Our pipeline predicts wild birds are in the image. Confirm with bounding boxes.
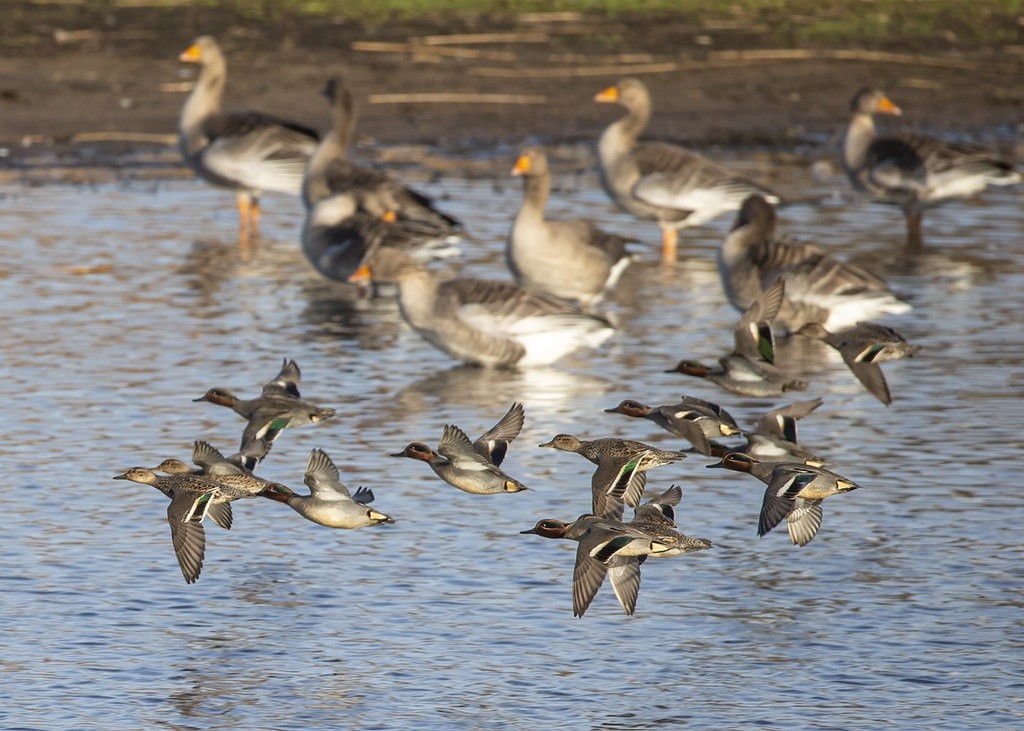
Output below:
[391,261,609,373]
[788,322,923,406]
[602,396,828,469]
[538,434,686,521]
[716,193,908,333]
[299,72,463,301]
[266,449,395,529]
[661,276,806,396]
[114,468,255,584]
[505,137,645,299]
[837,89,1020,248]
[150,442,266,530]
[705,451,861,548]
[594,80,782,265]
[192,358,334,462]
[390,401,536,495]
[171,34,326,235]
[606,484,714,617]
[517,515,673,618]
